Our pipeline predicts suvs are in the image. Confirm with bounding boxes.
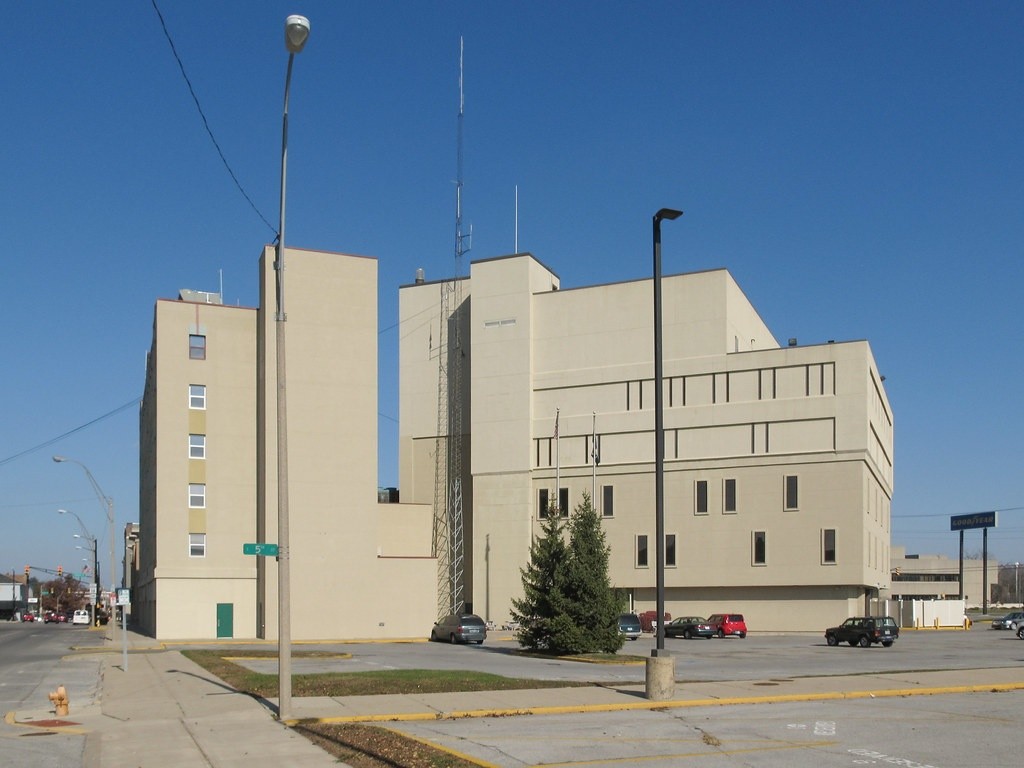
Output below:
[824,615,899,649]
[1001,612,1024,631]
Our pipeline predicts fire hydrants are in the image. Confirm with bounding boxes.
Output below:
[48,686,68,718]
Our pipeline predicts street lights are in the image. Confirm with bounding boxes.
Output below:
[52,454,117,645]
[57,508,98,631]
[638,206,684,685]
[276,14,313,727]
[1014,562,1020,603]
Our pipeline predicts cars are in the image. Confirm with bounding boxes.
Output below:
[1016,620,1024,641]
[34,611,69,625]
[616,612,643,641]
[431,614,487,644]
[23,613,34,622]
[665,617,719,639]
[991,617,1003,630]
[707,614,748,639]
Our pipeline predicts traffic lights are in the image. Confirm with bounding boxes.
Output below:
[57,566,62,576]
[24,566,29,576]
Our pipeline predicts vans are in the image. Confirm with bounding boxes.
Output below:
[72,609,90,626]
